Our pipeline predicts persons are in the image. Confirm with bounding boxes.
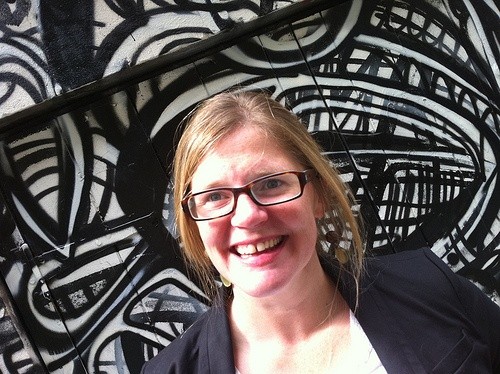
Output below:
[140,92,500,374]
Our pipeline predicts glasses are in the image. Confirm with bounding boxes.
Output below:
[180,168,319,220]
